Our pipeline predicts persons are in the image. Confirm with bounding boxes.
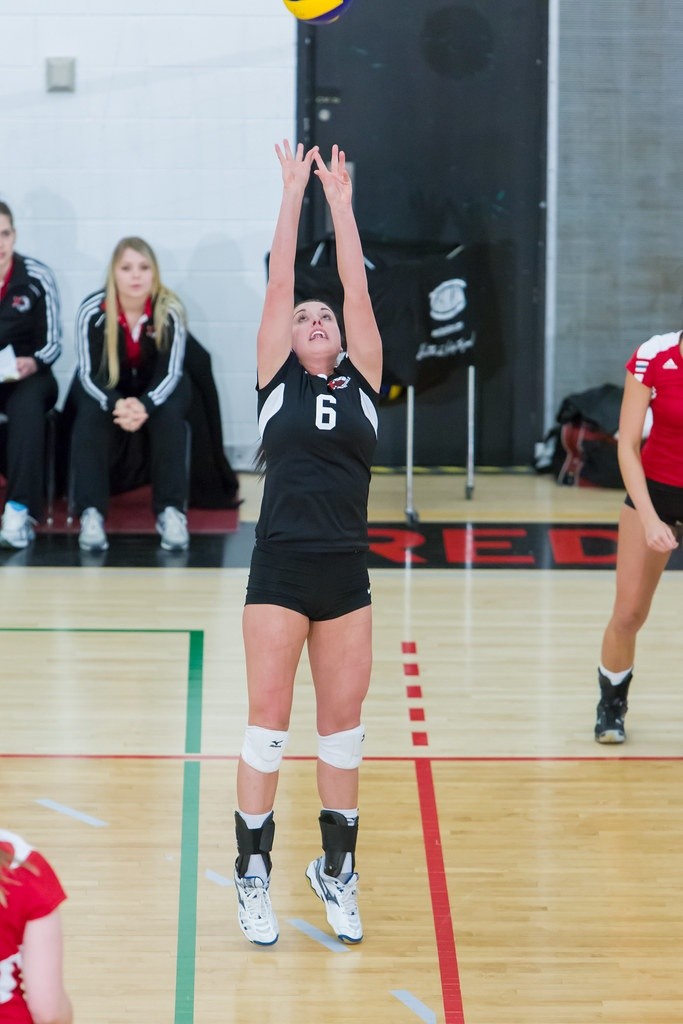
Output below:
[235,137,383,944]
[70,238,192,551]
[595,329,683,741]
[0,202,62,549]
[0,828,72,1024]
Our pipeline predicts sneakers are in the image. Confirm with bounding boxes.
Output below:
[233,865,280,946]
[594,700,628,744]
[0,501,37,549]
[304,855,364,945]
[155,506,190,553]
[78,506,110,553]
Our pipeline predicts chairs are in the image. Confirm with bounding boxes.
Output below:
[65,418,192,527]
[0,410,57,525]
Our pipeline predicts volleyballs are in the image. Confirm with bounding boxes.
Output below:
[280,0,353,28]
